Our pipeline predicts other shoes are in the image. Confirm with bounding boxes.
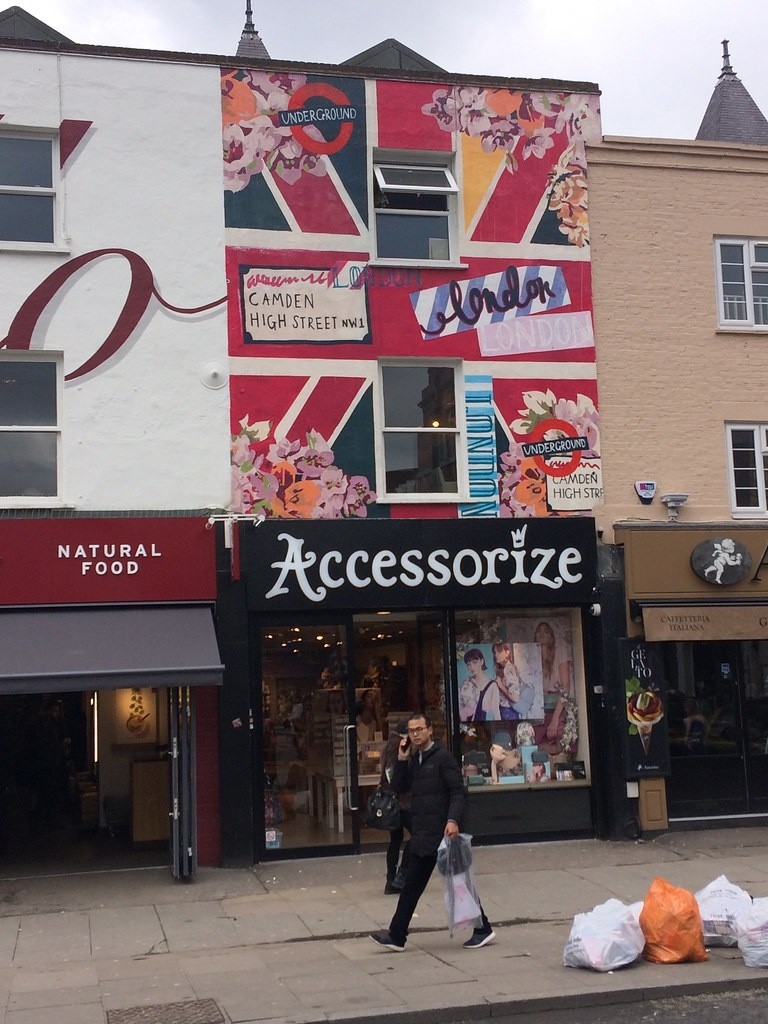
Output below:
[385,886,401,894]
[392,877,406,889]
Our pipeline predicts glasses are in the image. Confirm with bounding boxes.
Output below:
[406,727,428,734]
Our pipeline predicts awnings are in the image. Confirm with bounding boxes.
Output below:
[630,598,768,642]
[0,609,225,695]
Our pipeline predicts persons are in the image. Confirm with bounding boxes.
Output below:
[529,761,549,782]
[460,648,502,721]
[491,642,521,721]
[269,706,311,823]
[483,721,511,751]
[382,721,412,896]
[369,711,496,953]
[527,622,577,752]
[357,689,382,741]
[685,699,707,750]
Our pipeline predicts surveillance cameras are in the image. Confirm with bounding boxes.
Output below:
[588,603,601,617]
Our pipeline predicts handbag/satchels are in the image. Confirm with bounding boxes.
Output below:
[693,874,752,948]
[438,835,470,874]
[735,897,768,967]
[443,873,486,936]
[563,898,645,971]
[363,785,401,829]
[640,879,710,962]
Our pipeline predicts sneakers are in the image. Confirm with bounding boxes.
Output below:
[462,923,495,949]
[369,933,404,952]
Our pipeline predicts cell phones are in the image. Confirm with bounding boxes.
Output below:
[400,735,412,755]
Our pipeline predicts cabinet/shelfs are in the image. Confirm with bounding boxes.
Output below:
[269,688,442,833]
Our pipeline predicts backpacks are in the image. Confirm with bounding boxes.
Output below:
[508,682,535,714]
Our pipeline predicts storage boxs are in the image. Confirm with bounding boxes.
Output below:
[469,746,584,786]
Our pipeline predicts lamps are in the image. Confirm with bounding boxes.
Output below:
[660,492,691,519]
